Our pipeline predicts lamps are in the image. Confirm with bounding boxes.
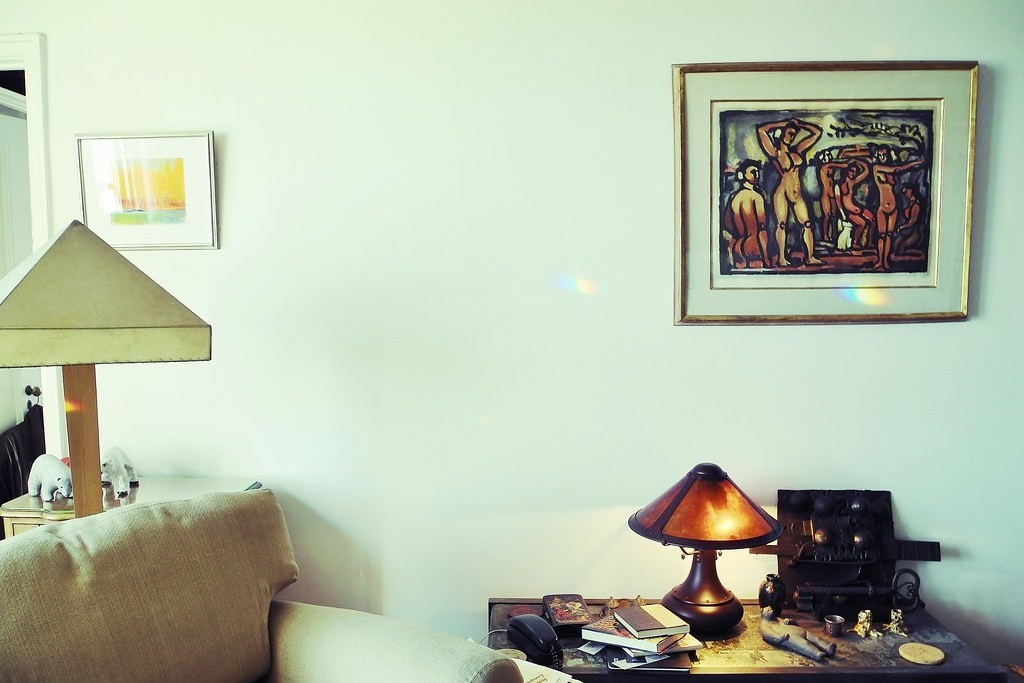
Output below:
[0,221,212,516]
[628,464,785,647]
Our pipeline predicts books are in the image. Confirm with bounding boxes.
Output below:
[619,627,703,658]
[613,603,690,640]
[604,641,694,672]
[580,615,689,656]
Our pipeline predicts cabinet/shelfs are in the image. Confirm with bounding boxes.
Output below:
[4,478,72,540]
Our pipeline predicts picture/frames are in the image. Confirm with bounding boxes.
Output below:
[671,57,981,331]
[73,132,224,254]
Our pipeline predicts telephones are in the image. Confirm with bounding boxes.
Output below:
[508,614,564,666]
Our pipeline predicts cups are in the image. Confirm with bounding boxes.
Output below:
[825,615,845,638]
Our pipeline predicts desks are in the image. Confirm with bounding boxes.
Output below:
[486,589,1011,683]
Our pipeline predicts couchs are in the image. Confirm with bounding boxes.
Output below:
[0,480,533,683]
